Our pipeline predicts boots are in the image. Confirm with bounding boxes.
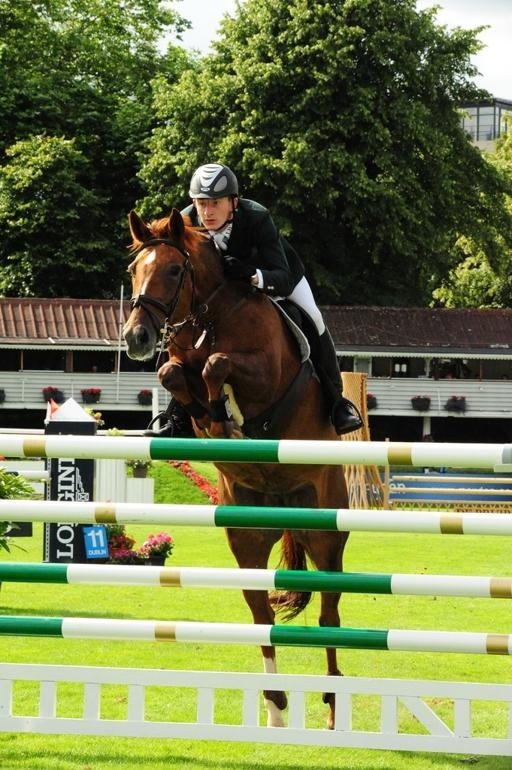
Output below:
[144,401,194,438]
[309,326,363,436]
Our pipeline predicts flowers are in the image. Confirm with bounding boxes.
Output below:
[108,525,176,564]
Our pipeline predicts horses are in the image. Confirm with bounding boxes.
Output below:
[122,207,351,730]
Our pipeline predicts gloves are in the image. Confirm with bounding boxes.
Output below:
[223,256,257,286]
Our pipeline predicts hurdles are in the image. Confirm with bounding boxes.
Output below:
[0,433,512,755]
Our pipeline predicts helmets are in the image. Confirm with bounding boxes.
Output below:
[188,162,239,200]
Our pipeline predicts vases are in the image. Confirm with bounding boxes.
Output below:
[145,555,166,566]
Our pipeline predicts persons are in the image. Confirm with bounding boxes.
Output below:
[140,163,362,439]
